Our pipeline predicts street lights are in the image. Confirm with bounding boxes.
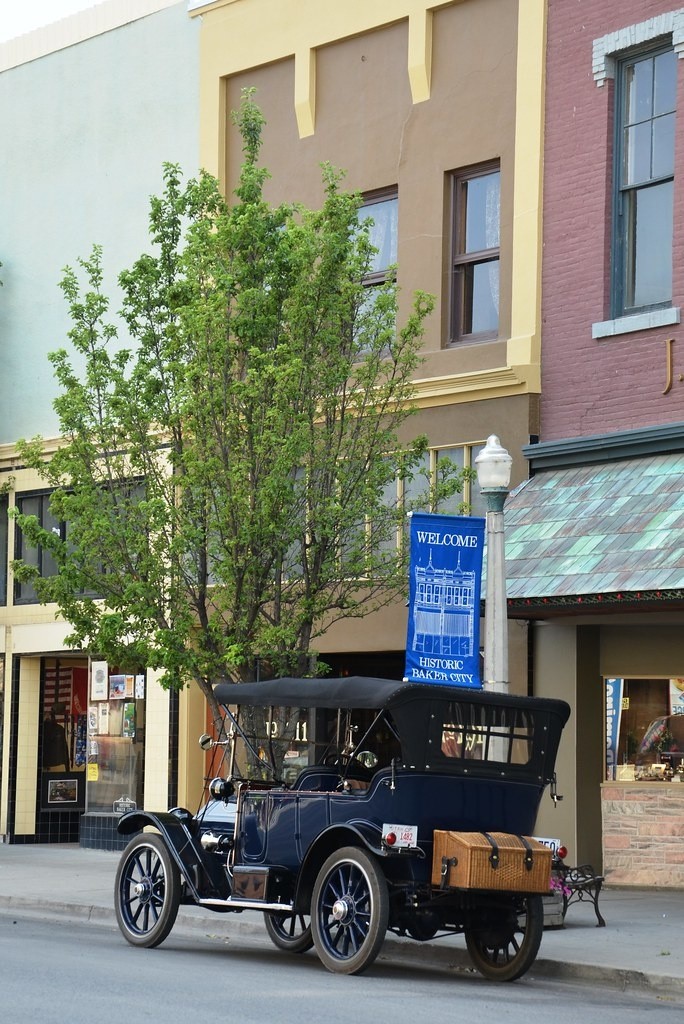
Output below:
[474,431,514,761]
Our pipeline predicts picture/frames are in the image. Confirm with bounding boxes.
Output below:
[48,780,78,803]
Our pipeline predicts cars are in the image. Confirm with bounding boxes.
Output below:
[113,675,572,983]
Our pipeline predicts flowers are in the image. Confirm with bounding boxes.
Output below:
[546,875,571,896]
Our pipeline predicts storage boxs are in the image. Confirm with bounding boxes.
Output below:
[432,828,553,894]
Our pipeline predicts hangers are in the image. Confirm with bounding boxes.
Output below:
[44,711,52,722]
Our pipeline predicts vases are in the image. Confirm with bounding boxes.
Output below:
[517,893,567,933]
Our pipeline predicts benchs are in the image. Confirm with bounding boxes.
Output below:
[290,765,377,792]
[551,857,606,930]
